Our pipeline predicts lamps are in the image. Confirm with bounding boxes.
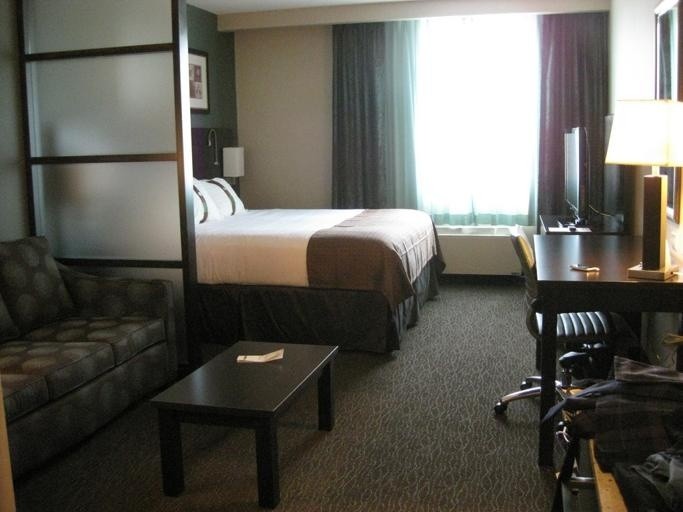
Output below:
[603,99,683,279]
[206,127,245,186]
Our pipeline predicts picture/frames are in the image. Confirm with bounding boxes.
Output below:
[187,47,210,114]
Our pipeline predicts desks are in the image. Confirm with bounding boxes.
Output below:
[524,233,683,468]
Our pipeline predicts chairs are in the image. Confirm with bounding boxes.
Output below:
[494,228,633,422]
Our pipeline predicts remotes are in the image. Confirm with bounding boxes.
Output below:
[568,225,576,231]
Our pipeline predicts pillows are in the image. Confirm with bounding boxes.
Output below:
[192,175,246,220]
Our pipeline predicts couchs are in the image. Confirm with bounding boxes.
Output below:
[0,235,180,484]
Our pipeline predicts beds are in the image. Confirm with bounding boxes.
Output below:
[192,209,445,355]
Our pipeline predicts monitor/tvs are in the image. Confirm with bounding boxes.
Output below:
[556,125,590,227]
